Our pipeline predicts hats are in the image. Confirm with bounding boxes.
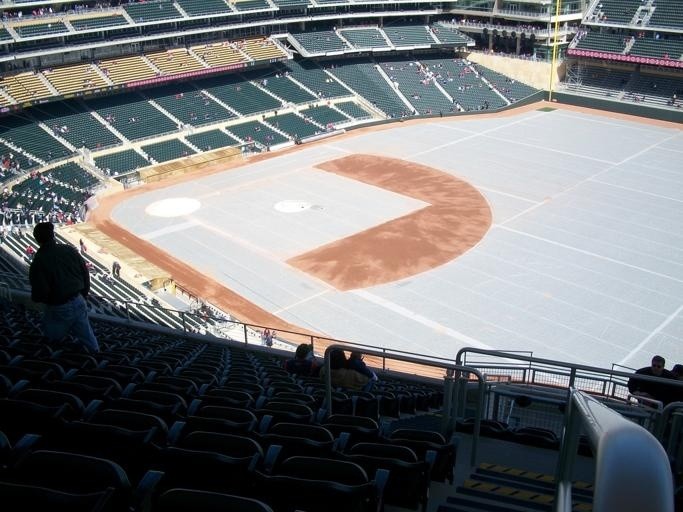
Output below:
[296,344,313,357]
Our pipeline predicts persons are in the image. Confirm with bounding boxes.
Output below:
[669,364,682,383]
[263,327,269,336]
[28,222,99,353]
[346,351,377,380]
[284,344,320,375]
[271,331,276,338]
[103,271,114,285]
[0,2,683,145]
[318,347,368,390]
[628,355,681,410]
[264,334,272,348]
[0,145,213,277]
